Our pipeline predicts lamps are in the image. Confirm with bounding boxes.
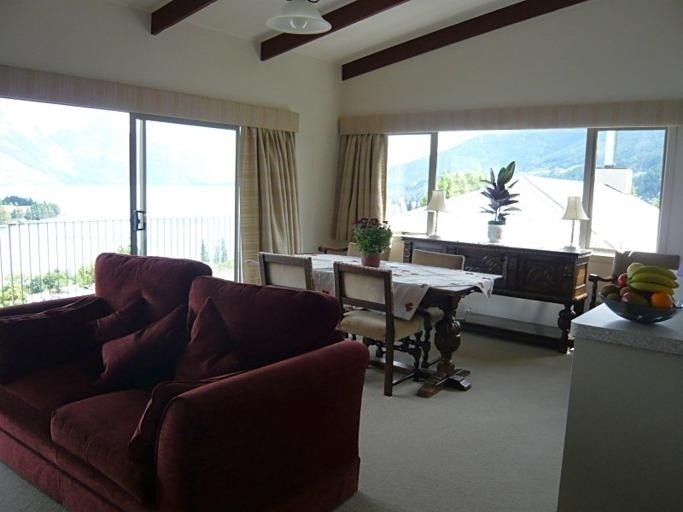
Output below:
[562,196,590,250]
[265,0,332,36]
[424,190,449,239]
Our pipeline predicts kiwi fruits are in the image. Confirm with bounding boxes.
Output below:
[619,287,632,297]
[600,285,619,297]
[607,293,619,303]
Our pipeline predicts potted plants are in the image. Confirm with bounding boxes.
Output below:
[352,218,391,267]
[479,161,521,244]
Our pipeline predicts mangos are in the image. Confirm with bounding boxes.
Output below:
[621,291,650,307]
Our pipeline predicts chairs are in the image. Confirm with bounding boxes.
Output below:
[348,242,404,262]
[588,250,680,309]
[376,250,465,368]
[258,252,315,293]
[100,303,188,391]
[81,298,143,373]
[333,261,425,396]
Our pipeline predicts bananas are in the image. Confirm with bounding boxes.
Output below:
[626,262,680,295]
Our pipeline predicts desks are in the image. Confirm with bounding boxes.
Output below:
[296,254,504,399]
[400,235,591,354]
[557,303,683,512]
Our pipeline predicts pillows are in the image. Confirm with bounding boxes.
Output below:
[176,297,244,379]
[126,370,246,462]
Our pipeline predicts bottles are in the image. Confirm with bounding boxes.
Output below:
[673,261,683,309]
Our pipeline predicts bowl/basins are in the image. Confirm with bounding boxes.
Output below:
[598,292,677,325]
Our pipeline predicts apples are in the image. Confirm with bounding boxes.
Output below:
[618,273,627,287]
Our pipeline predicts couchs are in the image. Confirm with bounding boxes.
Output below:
[0,253,369,511]
